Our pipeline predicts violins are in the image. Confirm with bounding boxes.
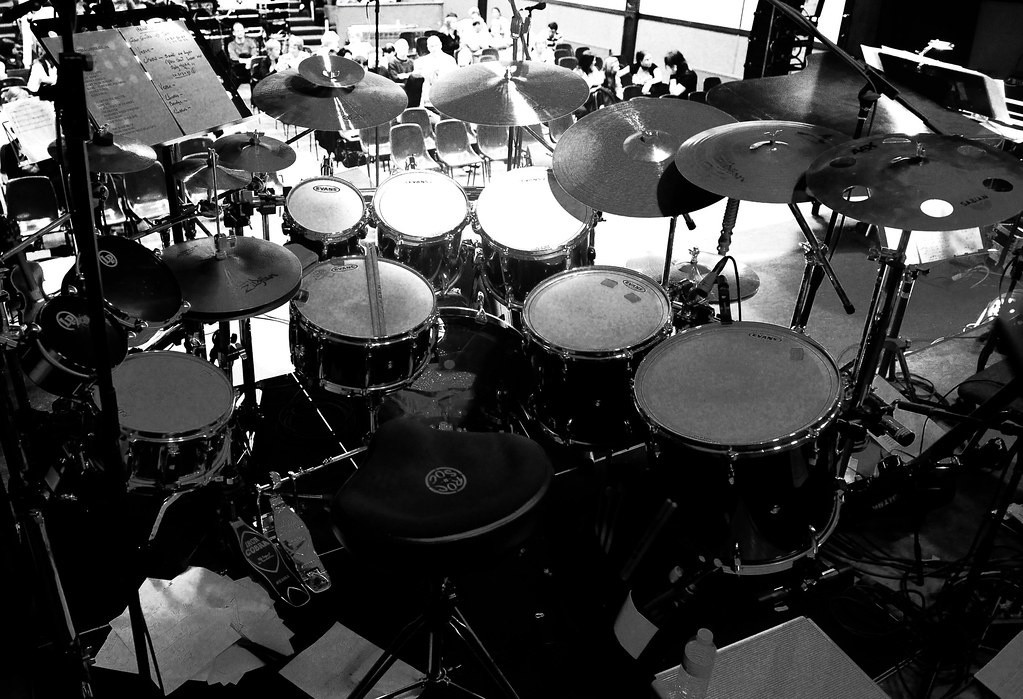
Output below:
[3,215,51,325]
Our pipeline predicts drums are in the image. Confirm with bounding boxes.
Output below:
[305,292,555,544]
[86,349,235,498]
[287,254,440,398]
[632,319,844,576]
[64,233,186,348]
[19,291,129,400]
[519,264,672,447]
[370,169,471,296]
[283,176,367,261]
[475,163,597,314]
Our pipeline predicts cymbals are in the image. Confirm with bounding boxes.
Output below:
[211,132,298,173]
[712,52,1006,150]
[546,95,740,219]
[49,131,159,175]
[805,129,1023,234]
[250,54,409,133]
[433,60,592,128]
[168,157,253,191]
[624,250,762,305]
[160,234,302,324]
[672,118,853,205]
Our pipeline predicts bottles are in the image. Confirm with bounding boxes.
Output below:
[675,628,717,699]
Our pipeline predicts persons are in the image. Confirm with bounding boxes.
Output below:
[5,31,57,103]
[228,7,565,89]
[572,50,697,100]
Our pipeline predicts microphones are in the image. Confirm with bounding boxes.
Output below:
[695,257,728,297]
[520,2,546,11]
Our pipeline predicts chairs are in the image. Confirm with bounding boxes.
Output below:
[0,31,720,240]
[974,628,1022,699]
